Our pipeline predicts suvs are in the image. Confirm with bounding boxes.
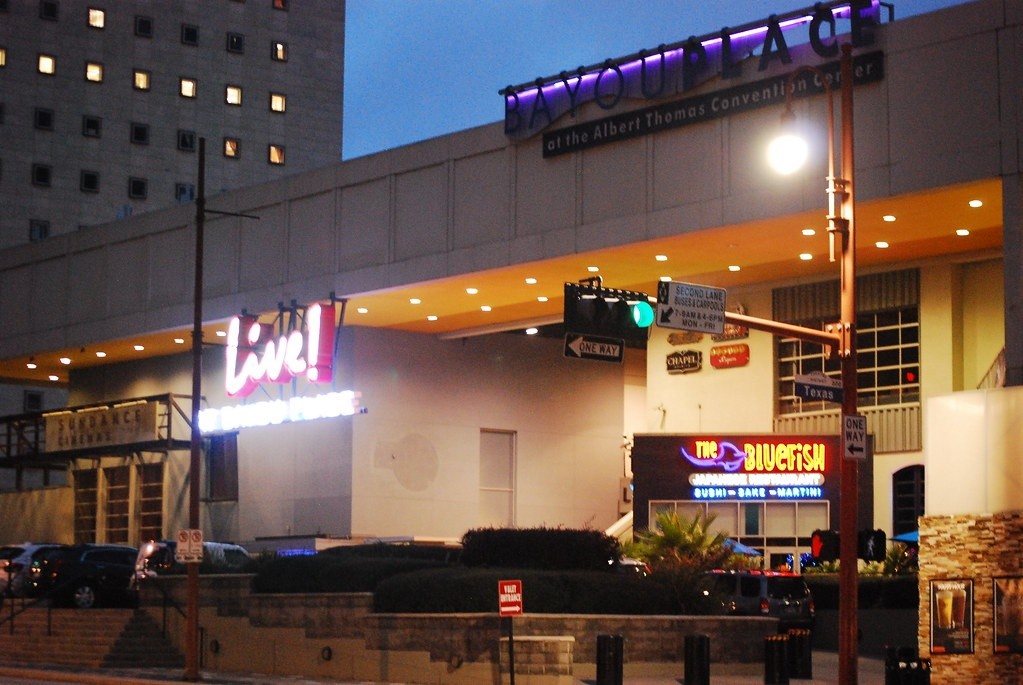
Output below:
[30,542,142,609]
[695,567,818,645]
[0,542,63,597]
[128,540,255,600]
[616,555,654,588]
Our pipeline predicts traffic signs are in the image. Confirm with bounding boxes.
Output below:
[498,579,523,617]
[561,331,626,363]
[654,279,726,334]
[841,414,868,460]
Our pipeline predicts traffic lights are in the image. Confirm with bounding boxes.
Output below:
[563,281,655,348]
[857,526,888,562]
[809,528,840,562]
